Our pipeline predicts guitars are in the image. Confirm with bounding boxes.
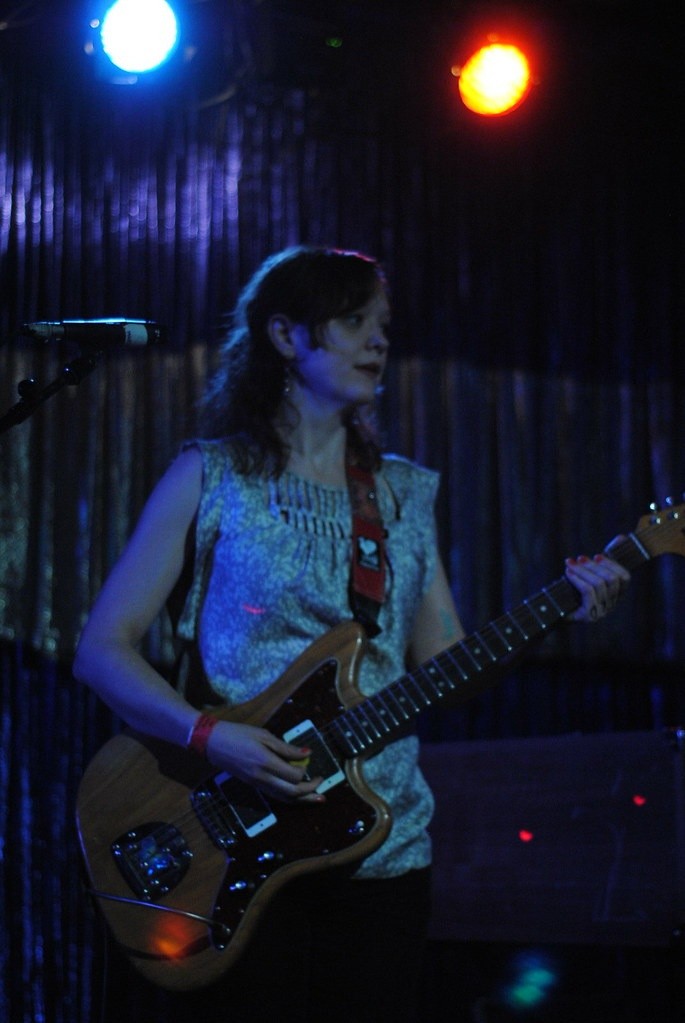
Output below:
[75,492,685,995]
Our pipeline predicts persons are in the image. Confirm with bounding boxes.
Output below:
[72,244,636,1023]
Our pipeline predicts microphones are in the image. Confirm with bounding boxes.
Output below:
[24,319,170,347]
[88,888,232,938]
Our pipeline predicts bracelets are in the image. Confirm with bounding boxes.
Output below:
[188,713,219,756]
